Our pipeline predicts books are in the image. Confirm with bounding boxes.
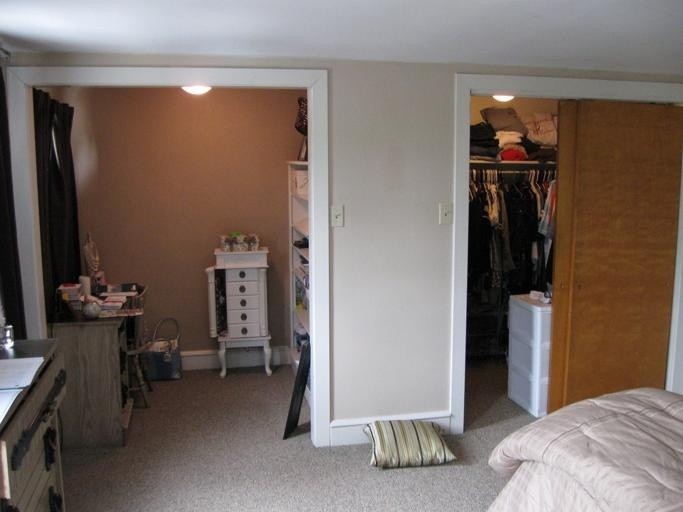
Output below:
[54,283,86,310]
[99,296,126,310]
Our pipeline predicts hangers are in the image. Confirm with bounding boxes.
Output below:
[468,167,555,199]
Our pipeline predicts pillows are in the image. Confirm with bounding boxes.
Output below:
[363,417,457,471]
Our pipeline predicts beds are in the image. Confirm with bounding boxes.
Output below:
[485,386,681,512]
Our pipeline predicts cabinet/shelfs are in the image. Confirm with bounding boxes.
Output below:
[283,159,309,401]
[53,281,138,446]
[205,248,275,379]
[0,330,68,511]
[505,290,550,419]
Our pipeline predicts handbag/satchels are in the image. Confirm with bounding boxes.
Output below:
[141,338,182,380]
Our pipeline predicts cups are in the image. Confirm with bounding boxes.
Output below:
[0,325,14,350]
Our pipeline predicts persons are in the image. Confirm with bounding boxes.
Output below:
[82,232,100,277]
